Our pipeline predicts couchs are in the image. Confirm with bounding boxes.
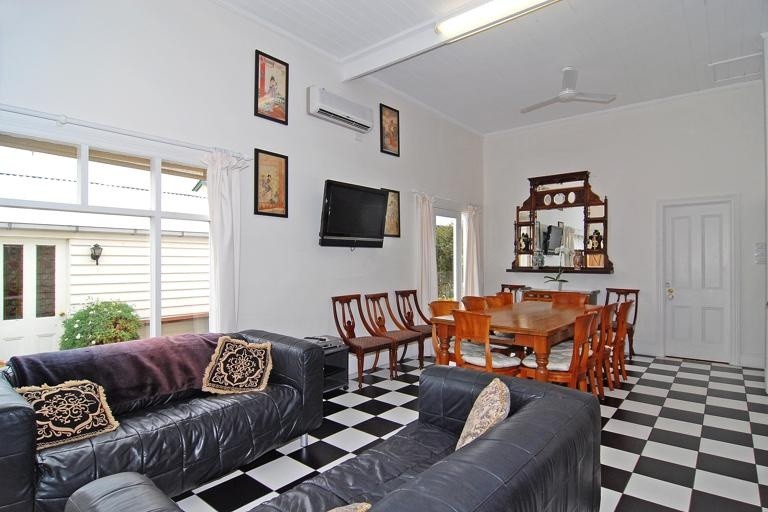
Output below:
[0,328,326,511]
[62,363,602,512]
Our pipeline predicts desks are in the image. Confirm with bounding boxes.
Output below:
[522,288,591,305]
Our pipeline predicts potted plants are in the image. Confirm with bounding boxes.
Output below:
[543,267,569,291]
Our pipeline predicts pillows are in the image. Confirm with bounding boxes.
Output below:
[326,500,374,512]
[11,378,120,452]
[454,377,512,453]
[200,335,273,395]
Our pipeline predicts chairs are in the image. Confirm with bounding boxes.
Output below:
[484,295,505,309]
[519,311,595,393]
[588,302,618,392]
[548,292,590,307]
[452,308,522,376]
[501,283,525,304]
[364,293,425,379]
[428,300,494,365]
[551,306,606,399]
[331,293,395,388]
[597,299,634,380]
[462,295,487,313]
[497,291,514,309]
[597,287,641,361]
[395,288,433,369]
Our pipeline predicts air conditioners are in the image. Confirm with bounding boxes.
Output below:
[306,84,373,135]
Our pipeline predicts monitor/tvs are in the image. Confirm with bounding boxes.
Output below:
[545,225,563,255]
[319,179,389,248]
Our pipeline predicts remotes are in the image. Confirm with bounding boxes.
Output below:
[304,335,326,342]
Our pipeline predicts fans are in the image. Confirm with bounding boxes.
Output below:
[519,67,617,115]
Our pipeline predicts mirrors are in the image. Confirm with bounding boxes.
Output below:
[506,169,616,274]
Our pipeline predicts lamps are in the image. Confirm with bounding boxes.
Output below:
[90,242,104,266]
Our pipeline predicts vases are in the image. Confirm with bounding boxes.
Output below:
[532,250,545,271]
[573,249,585,271]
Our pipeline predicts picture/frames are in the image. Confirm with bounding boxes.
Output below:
[254,48,290,128]
[378,102,402,158]
[253,147,289,219]
[380,187,402,240]
